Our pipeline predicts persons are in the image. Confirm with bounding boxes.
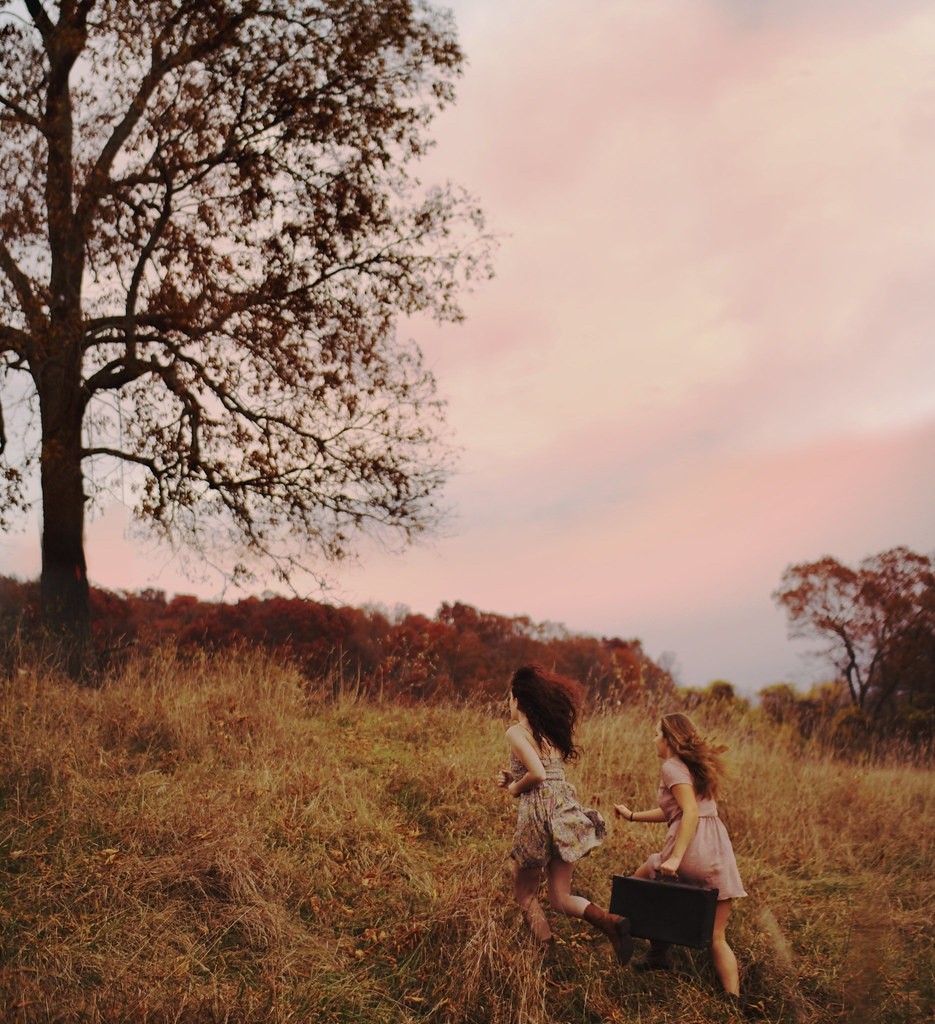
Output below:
[613,713,748,1009]
[497,665,632,988]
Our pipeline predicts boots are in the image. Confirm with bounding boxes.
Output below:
[539,935,562,965]
[584,902,634,967]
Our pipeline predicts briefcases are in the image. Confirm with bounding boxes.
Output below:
[609,869,720,950]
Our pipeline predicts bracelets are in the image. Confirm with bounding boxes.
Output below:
[631,812,634,822]
